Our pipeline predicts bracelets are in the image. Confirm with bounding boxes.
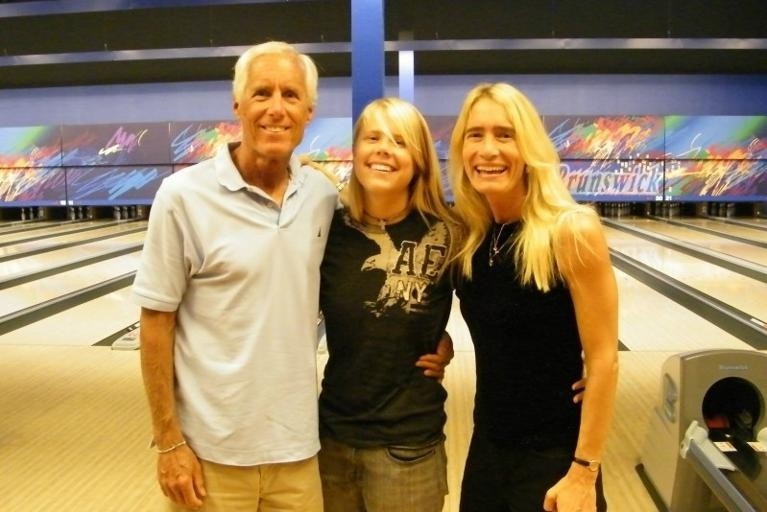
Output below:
[155,440,188,455]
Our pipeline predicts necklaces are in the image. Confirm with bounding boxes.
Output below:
[486,215,523,269]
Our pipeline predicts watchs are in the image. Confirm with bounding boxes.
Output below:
[571,455,600,472]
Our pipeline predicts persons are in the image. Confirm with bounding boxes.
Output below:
[316,98,589,512]
[442,82,622,512]
[130,39,455,512]
